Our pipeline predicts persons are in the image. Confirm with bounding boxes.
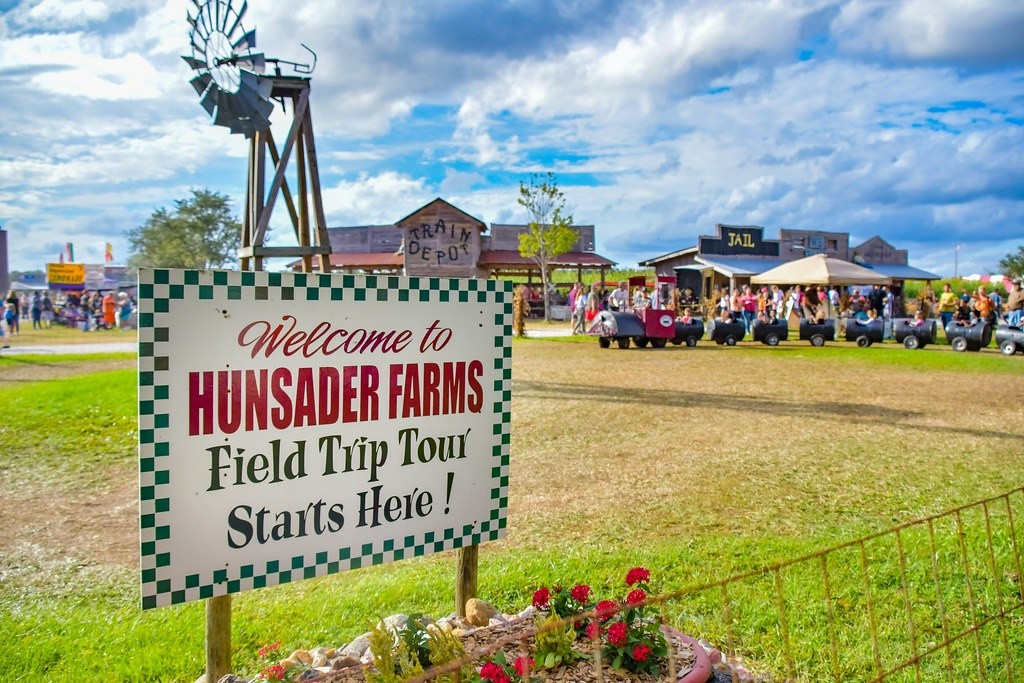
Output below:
[520,279,1022,348]
[0,289,132,339]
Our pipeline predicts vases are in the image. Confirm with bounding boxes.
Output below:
[305,618,711,683]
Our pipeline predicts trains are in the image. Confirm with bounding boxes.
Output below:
[584,304,1024,356]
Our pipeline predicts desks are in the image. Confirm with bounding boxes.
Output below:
[550,305,570,322]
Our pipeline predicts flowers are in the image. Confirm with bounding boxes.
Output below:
[255,567,668,683]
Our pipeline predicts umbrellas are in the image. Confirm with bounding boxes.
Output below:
[750,251,891,318]
